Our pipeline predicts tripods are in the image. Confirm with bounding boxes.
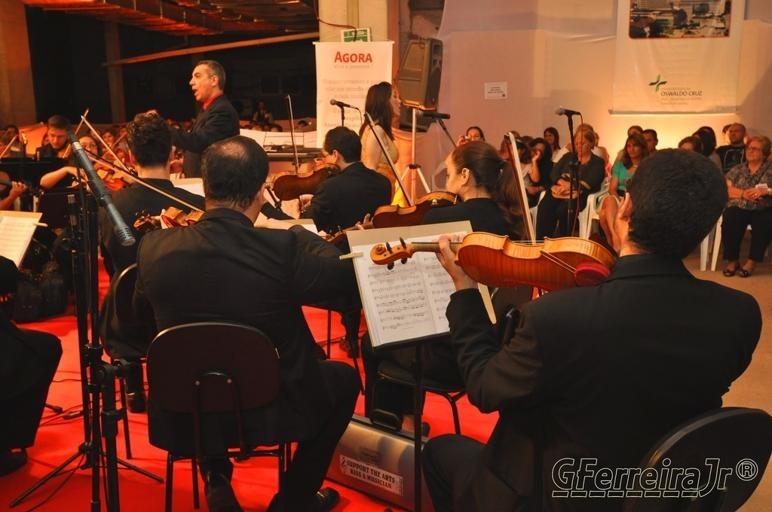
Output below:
[9,246,166,508]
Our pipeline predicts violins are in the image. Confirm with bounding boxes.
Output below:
[319,190,462,244]
[132,206,206,230]
[370,230,614,292]
[264,163,340,200]
[86,161,136,193]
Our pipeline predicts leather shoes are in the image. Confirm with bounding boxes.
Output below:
[738,267,751,276]
[206,471,244,512]
[0,452,27,475]
[265,487,339,510]
[123,389,146,412]
[723,268,736,276]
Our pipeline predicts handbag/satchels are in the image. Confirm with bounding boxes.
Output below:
[551,185,583,200]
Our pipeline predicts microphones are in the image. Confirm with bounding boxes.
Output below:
[330,99,356,111]
[555,108,580,117]
[416,109,452,120]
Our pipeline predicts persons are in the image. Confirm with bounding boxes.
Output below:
[453,120,772,279]
[260,126,393,358]
[357,80,403,203]
[128,131,364,510]
[359,139,522,437]
[669,2,689,30]
[420,147,763,512]
[0,99,313,317]
[1,255,65,479]
[142,57,242,178]
